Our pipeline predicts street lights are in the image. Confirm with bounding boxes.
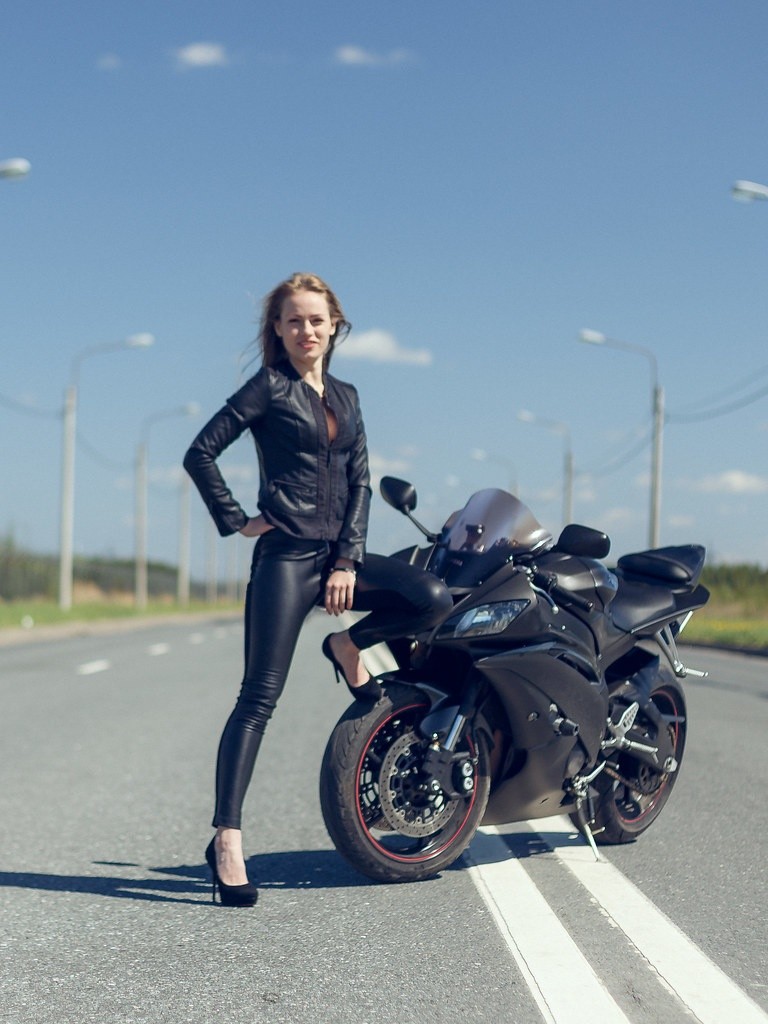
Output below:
[56,330,153,611]
[468,443,519,502]
[642,327,664,576]
[510,413,574,531]
[136,403,198,614]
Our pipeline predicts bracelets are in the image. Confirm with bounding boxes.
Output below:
[329,568,357,584]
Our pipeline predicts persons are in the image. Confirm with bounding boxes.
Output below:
[184,272,453,907]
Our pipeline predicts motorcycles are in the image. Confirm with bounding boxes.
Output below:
[315,476,714,884]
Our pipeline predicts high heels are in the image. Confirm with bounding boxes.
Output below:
[205,835,258,907]
[322,632,382,704]
